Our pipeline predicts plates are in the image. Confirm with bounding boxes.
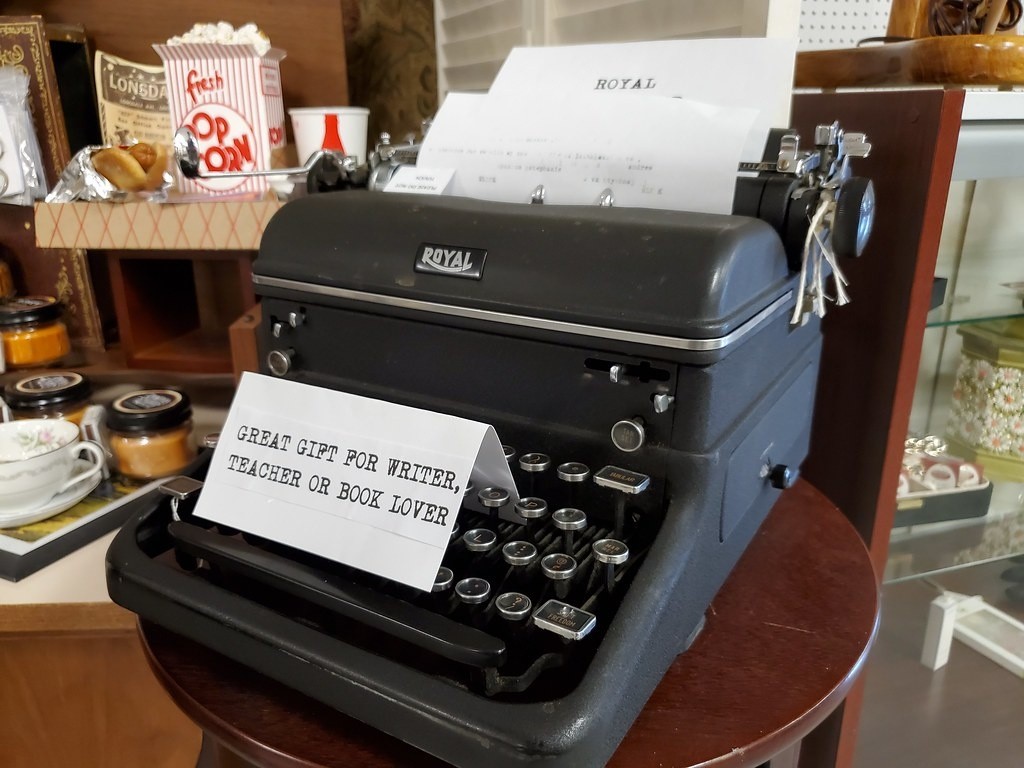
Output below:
[0,458,103,529]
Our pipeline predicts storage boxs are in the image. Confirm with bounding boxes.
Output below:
[150,42,289,195]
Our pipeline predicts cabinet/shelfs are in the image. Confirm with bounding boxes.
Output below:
[790,86,1024,587]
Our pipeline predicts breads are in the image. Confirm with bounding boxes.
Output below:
[91,142,168,193]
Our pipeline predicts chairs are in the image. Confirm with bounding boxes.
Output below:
[136,84,968,768]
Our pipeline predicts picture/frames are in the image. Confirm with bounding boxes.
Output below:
[0,472,183,582]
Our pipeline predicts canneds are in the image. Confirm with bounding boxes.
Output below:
[0,296,198,482]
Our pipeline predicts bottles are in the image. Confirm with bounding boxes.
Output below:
[0,293,198,489]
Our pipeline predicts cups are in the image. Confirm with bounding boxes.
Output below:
[0,420,106,514]
[287,106,371,169]
